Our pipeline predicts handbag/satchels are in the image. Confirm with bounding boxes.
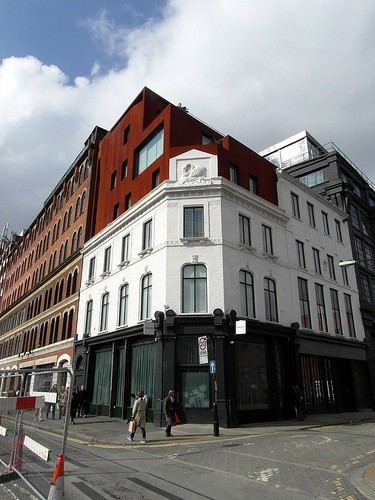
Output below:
[175,414,180,424]
[128,418,135,433]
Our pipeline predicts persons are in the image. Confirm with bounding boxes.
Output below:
[7,383,98,424]
[127,391,146,444]
[123,393,151,424]
[163,390,175,437]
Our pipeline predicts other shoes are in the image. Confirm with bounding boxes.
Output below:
[141,441,145,443]
[34,419,37,421]
[127,437,132,441]
[167,435,173,437]
[39,418,42,422]
